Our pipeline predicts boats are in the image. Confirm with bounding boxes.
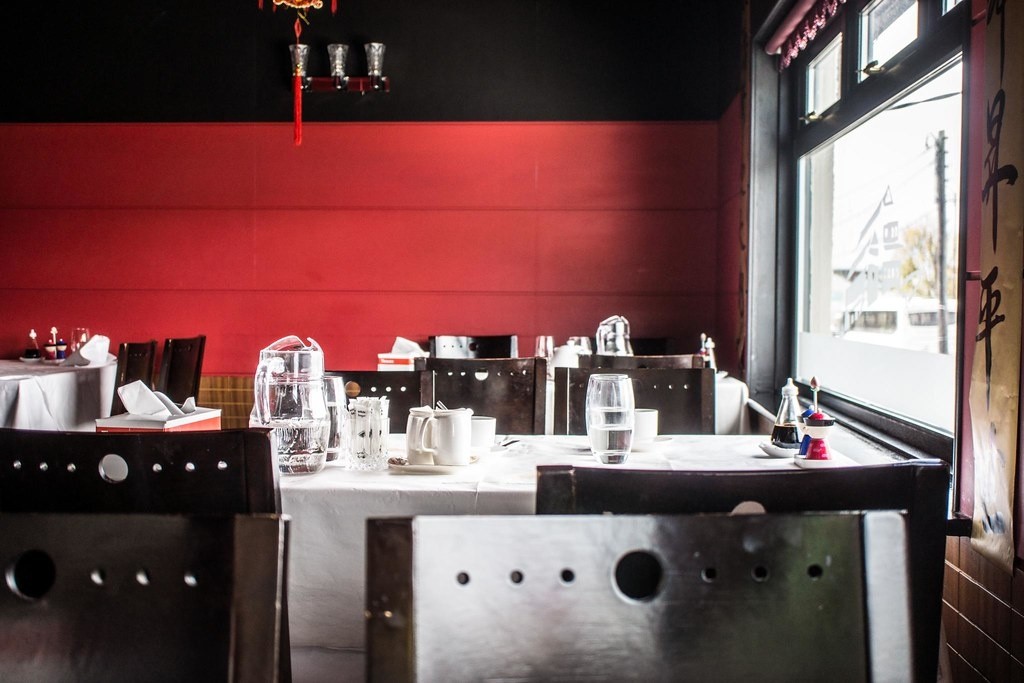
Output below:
[833,185,957,351]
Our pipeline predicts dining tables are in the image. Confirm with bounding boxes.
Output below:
[279,434,903,683]
[0,358,118,431]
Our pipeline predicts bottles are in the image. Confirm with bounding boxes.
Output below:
[770,377,806,449]
[24,328,41,358]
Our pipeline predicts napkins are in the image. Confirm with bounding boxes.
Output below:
[59,334,111,369]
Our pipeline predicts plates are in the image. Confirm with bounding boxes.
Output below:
[759,441,802,456]
[20,356,42,363]
[43,357,65,364]
[632,435,673,452]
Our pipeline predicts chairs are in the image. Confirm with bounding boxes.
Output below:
[325,332,752,432]
[109,340,158,416]
[156,333,207,408]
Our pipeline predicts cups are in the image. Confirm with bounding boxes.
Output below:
[71,328,89,352]
[535,330,592,381]
[469,415,497,449]
[585,373,657,463]
[405,407,436,464]
[318,372,347,462]
[342,412,391,471]
[419,405,473,466]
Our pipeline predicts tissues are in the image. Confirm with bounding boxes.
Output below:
[378,336,432,372]
[94,380,222,432]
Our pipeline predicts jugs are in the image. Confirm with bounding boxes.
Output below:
[255,334,331,476]
[596,313,637,355]
